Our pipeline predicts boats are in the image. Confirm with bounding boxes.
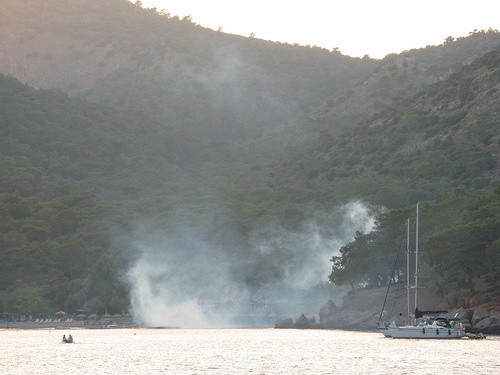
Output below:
[375,203,466,338]
